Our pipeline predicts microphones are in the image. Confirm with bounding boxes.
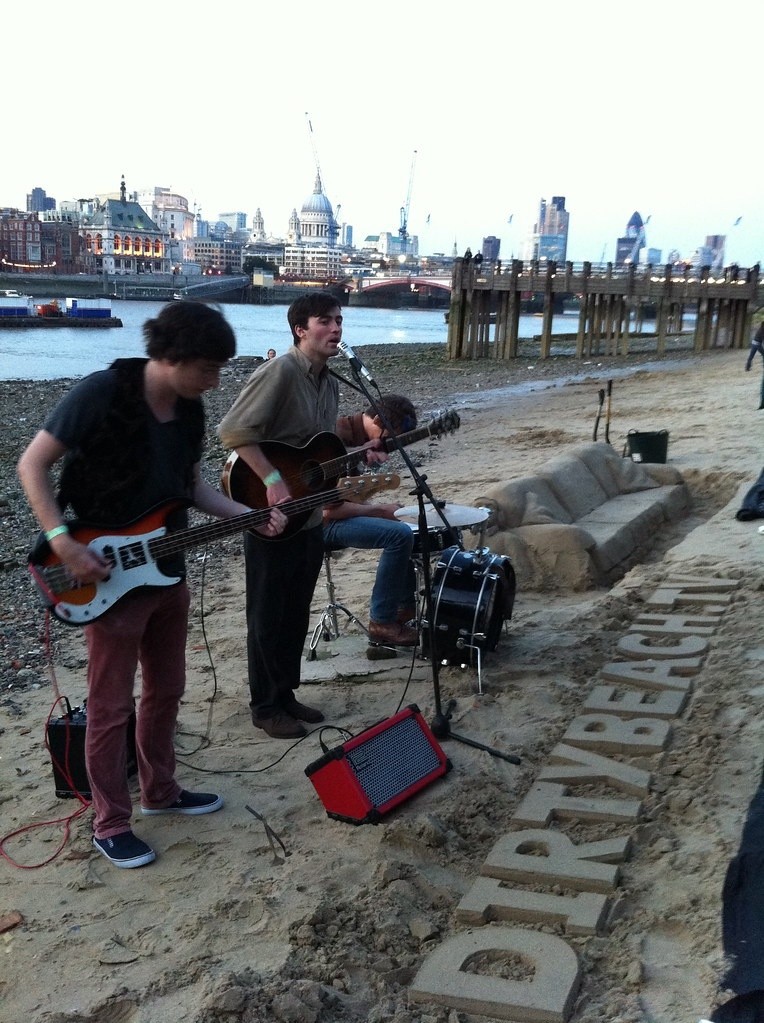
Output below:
[336,341,378,389]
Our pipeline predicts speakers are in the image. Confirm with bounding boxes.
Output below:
[47,704,139,800]
[304,703,454,826]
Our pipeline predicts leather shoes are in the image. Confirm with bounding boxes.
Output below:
[369,621,420,646]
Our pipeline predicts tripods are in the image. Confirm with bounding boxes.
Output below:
[351,368,522,765]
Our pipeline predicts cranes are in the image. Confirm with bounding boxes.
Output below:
[304,110,343,221]
[398,150,419,255]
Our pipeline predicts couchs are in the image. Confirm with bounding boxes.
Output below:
[474,442,688,588]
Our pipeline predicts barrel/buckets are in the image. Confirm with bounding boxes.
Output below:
[626,428,669,464]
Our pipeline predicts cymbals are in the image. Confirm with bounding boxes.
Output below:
[393,502,489,528]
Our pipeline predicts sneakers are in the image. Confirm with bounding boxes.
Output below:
[94,832,156,867]
[142,789,223,816]
[281,699,324,723]
[253,710,309,737]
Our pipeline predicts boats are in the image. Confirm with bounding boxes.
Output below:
[3,289,34,299]
[0,295,124,329]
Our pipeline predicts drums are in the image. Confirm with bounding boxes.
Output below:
[400,520,462,557]
[419,544,517,669]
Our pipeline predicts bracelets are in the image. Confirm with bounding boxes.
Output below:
[264,471,281,486]
[45,524,69,541]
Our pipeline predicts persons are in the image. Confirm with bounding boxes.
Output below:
[746,324,764,409]
[323,394,419,646]
[19,300,287,865]
[218,293,388,738]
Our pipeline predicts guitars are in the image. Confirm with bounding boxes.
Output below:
[223,406,464,541]
[25,468,401,627]
